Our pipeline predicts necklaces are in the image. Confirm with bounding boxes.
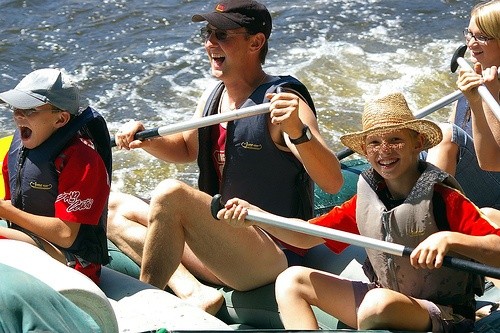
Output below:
[223,74,267,111]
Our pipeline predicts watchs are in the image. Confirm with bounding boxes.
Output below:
[288,125,313,145]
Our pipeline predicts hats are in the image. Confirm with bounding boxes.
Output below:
[340,92,443,156]
[191,0,272,39]
[0,67,80,117]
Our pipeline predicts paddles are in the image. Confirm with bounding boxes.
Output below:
[110,86,287,148]
[336,86,463,163]
[449,43,499,123]
[209,193,500,281]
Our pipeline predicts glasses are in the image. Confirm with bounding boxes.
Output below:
[462,27,496,43]
[199,26,251,40]
[8,101,63,115]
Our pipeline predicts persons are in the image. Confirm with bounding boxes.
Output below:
[475,207,500,321]
[419,0,500,210]
[0,68,110,285]
[456,62,500,173]
[216,93,500,333]
[106,0,343,317]
